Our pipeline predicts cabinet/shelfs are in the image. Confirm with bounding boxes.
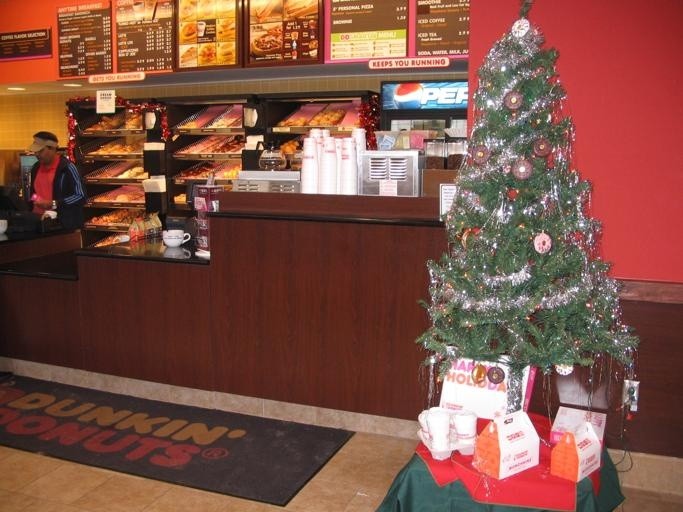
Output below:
[69,91,381,248]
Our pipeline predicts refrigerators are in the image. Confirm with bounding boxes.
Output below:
[380,79,468,137]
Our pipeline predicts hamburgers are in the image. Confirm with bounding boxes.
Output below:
[219,43,234,61]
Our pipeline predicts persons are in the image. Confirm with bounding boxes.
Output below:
[26,130,87,230]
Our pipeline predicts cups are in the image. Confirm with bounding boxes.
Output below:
[417,403,477,459]
[133,1,145,11]
[301,127,366,195]
[162,230,191,244]
[197,22,205,37]
[163,235,184,248]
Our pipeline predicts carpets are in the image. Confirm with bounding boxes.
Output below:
[0,367,354,506]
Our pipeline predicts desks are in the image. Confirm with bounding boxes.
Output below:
[412,412,617,511]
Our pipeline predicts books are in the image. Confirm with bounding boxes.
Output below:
[520,362,537,413]
[436,351,528,419]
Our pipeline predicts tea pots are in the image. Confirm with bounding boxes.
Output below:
[254,140,287,170]
[290,149,301,170]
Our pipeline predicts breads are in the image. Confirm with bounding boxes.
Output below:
[86,108,346,246]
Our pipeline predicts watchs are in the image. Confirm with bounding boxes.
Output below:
[50,199,57,211]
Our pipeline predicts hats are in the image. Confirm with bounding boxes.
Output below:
[26,136,58,152]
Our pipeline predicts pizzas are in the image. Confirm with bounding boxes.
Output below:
[252,34,282,56]
